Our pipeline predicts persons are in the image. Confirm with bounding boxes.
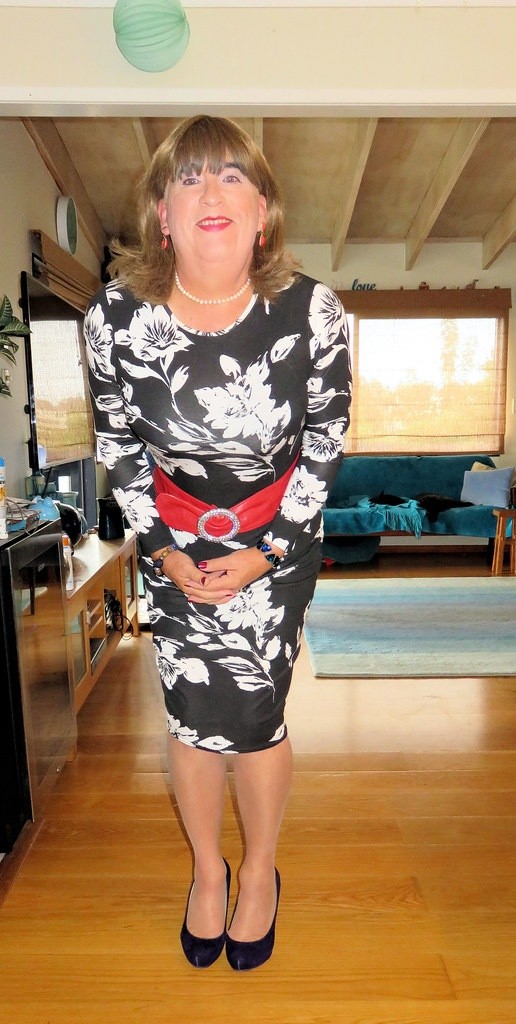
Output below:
[83,117,351,971]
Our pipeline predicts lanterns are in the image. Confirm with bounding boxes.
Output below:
[113,0,191,73]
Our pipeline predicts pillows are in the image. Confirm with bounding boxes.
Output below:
[470,460,495,470]
[460,466,516,509]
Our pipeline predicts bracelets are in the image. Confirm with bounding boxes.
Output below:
[256,539,281,570]
[154,542,178,577]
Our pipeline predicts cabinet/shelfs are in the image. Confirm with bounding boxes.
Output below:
[0,519,79,822]
[65,531,140,712]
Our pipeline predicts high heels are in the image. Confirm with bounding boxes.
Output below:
[180,856,231,967]
[226,866,281,970]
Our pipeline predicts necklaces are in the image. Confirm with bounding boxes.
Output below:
[171,262,252,304]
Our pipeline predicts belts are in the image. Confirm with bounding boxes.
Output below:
[152,447,300,541]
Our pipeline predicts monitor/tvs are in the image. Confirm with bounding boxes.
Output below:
[17,271,98,470]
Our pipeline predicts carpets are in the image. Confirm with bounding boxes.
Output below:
[306,577,516,678]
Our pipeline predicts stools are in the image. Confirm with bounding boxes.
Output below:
[491,509,516,576]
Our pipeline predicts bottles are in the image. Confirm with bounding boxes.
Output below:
[0,455,7,506]
[62,536,74,590]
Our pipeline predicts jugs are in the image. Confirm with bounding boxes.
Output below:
[96,497,126,540]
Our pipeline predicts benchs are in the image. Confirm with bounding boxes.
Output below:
[322,452,516,538]
